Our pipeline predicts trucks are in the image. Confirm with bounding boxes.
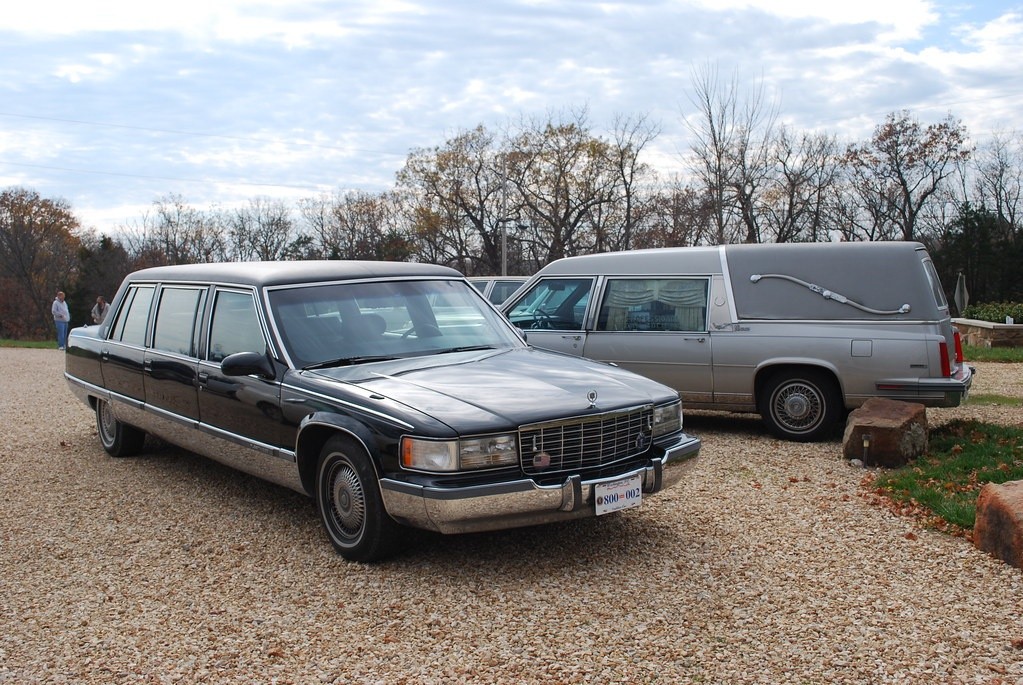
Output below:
[307,276,582,332]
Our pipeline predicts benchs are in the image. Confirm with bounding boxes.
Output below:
[278,317,343,345]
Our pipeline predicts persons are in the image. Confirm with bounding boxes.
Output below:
[52,292,70,350]
[91,296,112,325]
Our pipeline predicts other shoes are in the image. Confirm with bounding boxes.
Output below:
[59,346,67,349]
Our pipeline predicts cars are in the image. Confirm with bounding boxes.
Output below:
[63,260,701,563]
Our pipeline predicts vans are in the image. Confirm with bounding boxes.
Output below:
[381,241,976,443]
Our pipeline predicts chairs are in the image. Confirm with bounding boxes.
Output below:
[335,314,397,355]
